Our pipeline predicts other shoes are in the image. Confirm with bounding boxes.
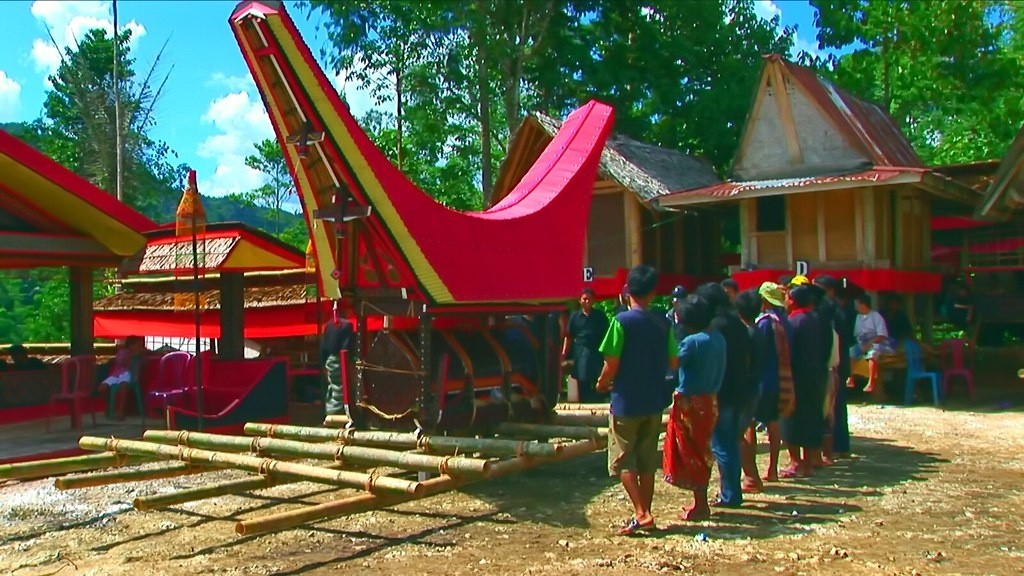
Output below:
[708,491,744,508]
[777,453,833,478]
[740,475,763,493]
[762,472,778,482]
[678,506,710,520]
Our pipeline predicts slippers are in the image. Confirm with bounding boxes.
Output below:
[615,511,656,535]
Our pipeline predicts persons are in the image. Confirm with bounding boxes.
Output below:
[99,334,148,421]
[321,308,357,417]
[561,263,974,537]
[9,345,49,405]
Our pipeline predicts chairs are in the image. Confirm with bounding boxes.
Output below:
[46,350,188,433]
[904,337,944,406]
[938,340,979,404]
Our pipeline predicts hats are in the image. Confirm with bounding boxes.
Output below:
[757,281,785,307]
[671,286,688,302]
[791,275,810,286]
